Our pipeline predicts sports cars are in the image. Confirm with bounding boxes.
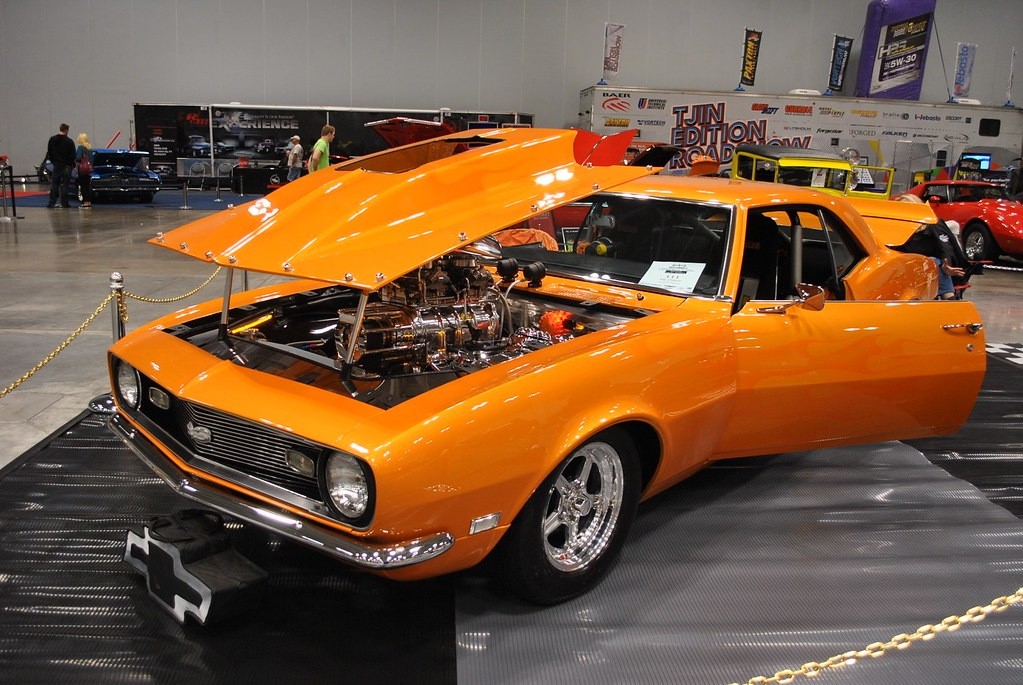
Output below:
[106,127,987,608]
[228,144,311,194]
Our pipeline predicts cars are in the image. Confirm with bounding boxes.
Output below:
[329,117,613,252]
[890,179,1023,265]
[256,138,315,158]
[181,135,211,158]
[58,148,162,203]
[38,159,78,185]
[154,165,176,176]
[730,144,897,200]
[213,141,235,156]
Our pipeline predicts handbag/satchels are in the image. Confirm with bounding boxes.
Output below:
[79,145,93,174]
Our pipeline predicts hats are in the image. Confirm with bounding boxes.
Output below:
[290,135,300,141]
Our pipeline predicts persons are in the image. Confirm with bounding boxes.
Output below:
[46,123,76,208]
[309,125,334,172]
[286,135,303,182]
[75,133,92,209]
[895,194,967,300]
[280,150,291,167]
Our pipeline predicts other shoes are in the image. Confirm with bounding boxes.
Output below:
[47,205,55,208]
[62,205,72,208]
[80,204,92,207]
